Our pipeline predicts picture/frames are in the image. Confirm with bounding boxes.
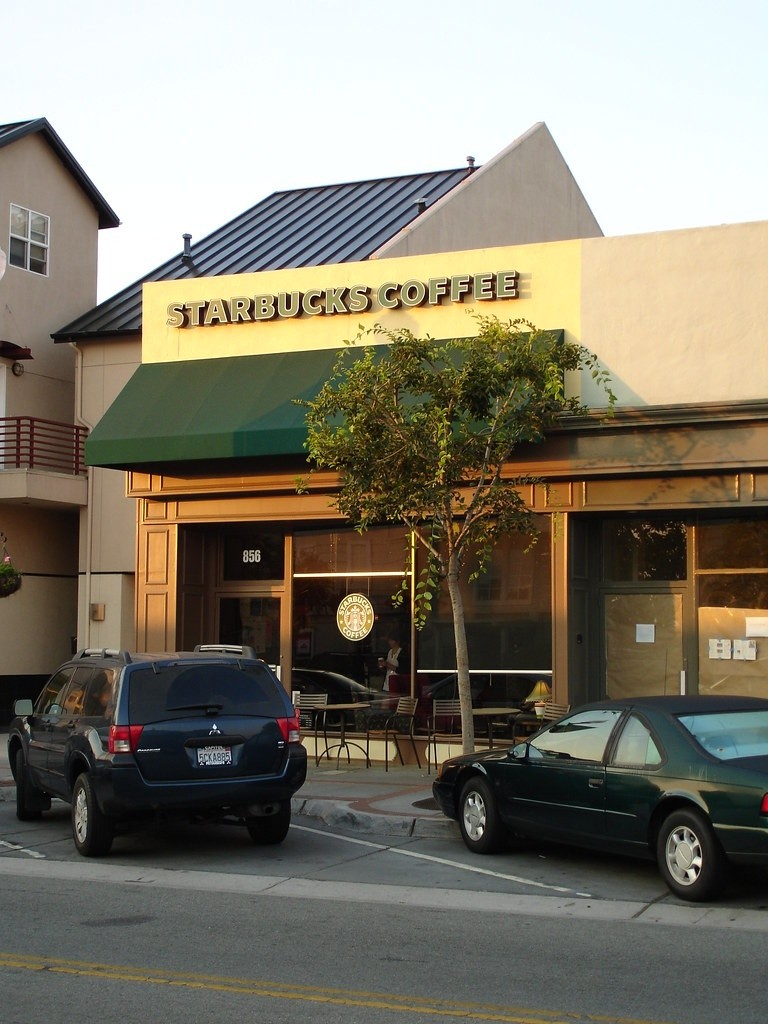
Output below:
[293,628,315,660]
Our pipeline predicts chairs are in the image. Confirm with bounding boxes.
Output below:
[426,699,462,775]
[366,697,422,772]
[294,694,329,764]
[513,701,570,748]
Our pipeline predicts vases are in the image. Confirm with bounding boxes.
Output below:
[0,576,22,598]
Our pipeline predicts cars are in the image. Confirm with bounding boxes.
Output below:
[432,695,768,902]
[268,664,404,731]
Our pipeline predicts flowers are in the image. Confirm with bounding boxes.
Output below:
[0,554,22,590]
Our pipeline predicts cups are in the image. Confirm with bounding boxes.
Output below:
[534,703,546,719]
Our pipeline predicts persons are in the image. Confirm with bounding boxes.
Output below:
[377,630,411,712]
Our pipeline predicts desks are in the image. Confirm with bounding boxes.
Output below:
[458,707,521,750]
[313,704,371,770]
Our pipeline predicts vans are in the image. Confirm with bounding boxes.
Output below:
[420,669,551,737]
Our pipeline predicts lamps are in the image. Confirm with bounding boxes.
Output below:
[524,680,552,719]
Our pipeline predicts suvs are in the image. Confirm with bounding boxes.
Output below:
[7,645,307,857]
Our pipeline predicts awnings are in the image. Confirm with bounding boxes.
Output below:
[86,327,569,477]
